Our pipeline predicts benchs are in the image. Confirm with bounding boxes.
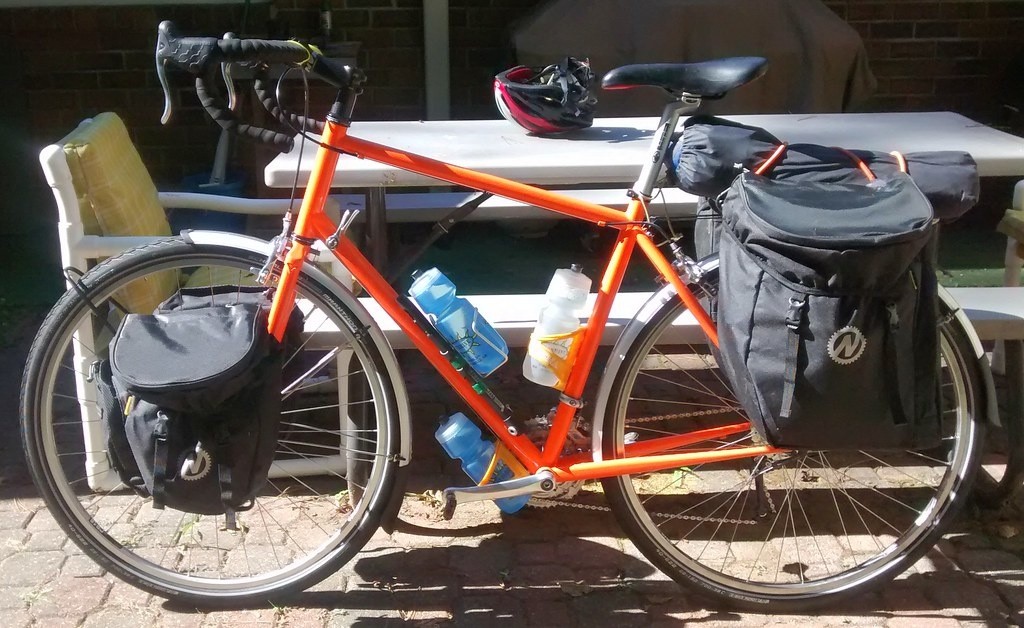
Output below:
[294,188,1024,522]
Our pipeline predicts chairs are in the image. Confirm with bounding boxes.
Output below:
[41,112,354,491]
[992,180,1024,376]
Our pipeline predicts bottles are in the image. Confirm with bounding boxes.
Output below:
[408,267,510,378]
[435,412,531,514]
[522,263,593,392]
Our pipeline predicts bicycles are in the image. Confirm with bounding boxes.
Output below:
[19,21,1004,614]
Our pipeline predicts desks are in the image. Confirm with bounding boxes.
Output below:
[265,111,1024,477]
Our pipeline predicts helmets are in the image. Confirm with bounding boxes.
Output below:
[494,57,598,135]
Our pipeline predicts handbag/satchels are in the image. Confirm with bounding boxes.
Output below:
[112,305,280,513]
[717,174,948,452]
[92,283,303,498]
[694,198,724,320]
